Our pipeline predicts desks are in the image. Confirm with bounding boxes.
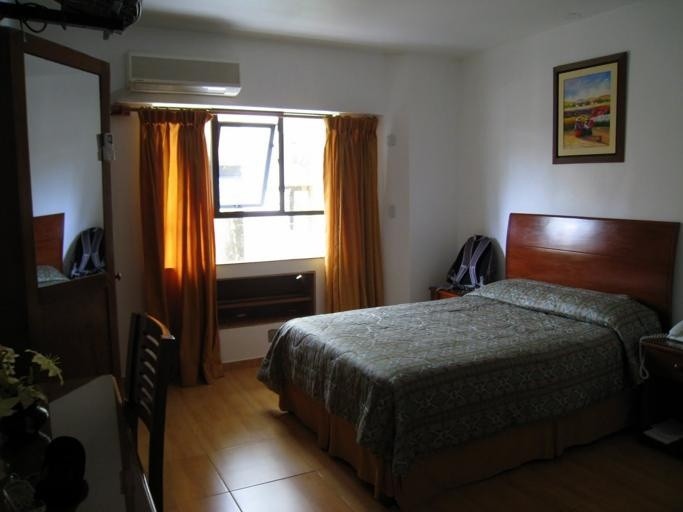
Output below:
[0,371,158,512]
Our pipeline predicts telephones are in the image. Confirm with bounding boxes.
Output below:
[667,320,683,342]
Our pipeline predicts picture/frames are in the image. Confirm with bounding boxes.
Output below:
[552,51,630,163]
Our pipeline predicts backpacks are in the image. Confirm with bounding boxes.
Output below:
[447,236,494,292]
[71,227,106,278]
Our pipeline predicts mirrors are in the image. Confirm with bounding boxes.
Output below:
[18,43,112,297]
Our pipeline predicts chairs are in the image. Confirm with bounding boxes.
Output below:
[120,309,175,512]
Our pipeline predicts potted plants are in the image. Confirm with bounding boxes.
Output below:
[0,346,66,448]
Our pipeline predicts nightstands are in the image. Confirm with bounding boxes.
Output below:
[431,284,460,299]
[642,336,682,435]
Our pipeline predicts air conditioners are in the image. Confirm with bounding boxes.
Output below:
[126,52,243,98]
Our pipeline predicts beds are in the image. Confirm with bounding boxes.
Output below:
[265,212,681,510]
